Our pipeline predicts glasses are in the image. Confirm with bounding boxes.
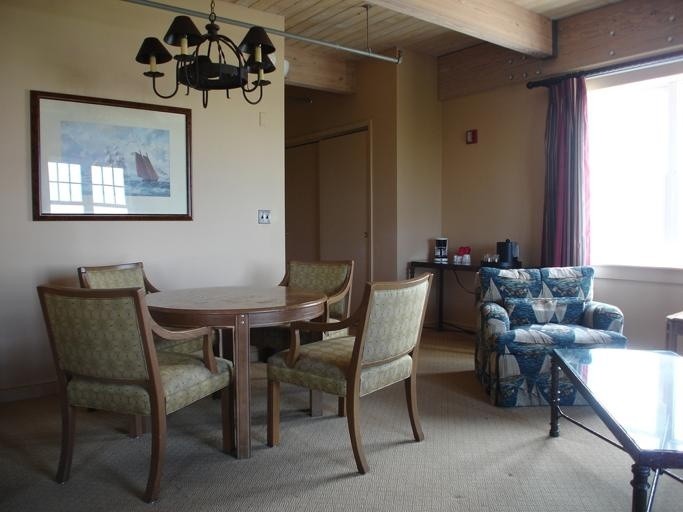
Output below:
[136,0,277,111]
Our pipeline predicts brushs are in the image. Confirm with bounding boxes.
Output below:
[432,237,448,264]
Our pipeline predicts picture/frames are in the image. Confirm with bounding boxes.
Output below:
[546,341,682,512]
[405,257,520,337]
[138,279,339,460]
[661,300,682,355]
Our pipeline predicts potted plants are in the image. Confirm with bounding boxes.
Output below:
[27,89,195,226]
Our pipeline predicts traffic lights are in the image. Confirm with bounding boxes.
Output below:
[503,293,587,328]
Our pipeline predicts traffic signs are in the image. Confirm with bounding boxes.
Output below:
[474,286,481,299]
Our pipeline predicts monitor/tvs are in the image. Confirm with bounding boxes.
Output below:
[496,242,520,264]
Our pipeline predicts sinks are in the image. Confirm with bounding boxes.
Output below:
[475,265,626,410]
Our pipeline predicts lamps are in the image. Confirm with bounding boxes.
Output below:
[36,282,240,505]
[252,258,354,419]
[264,271,434,475]
[77,262,228,440]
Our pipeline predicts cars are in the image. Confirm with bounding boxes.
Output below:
[453,254,470,266]
[481,253,500,263]
